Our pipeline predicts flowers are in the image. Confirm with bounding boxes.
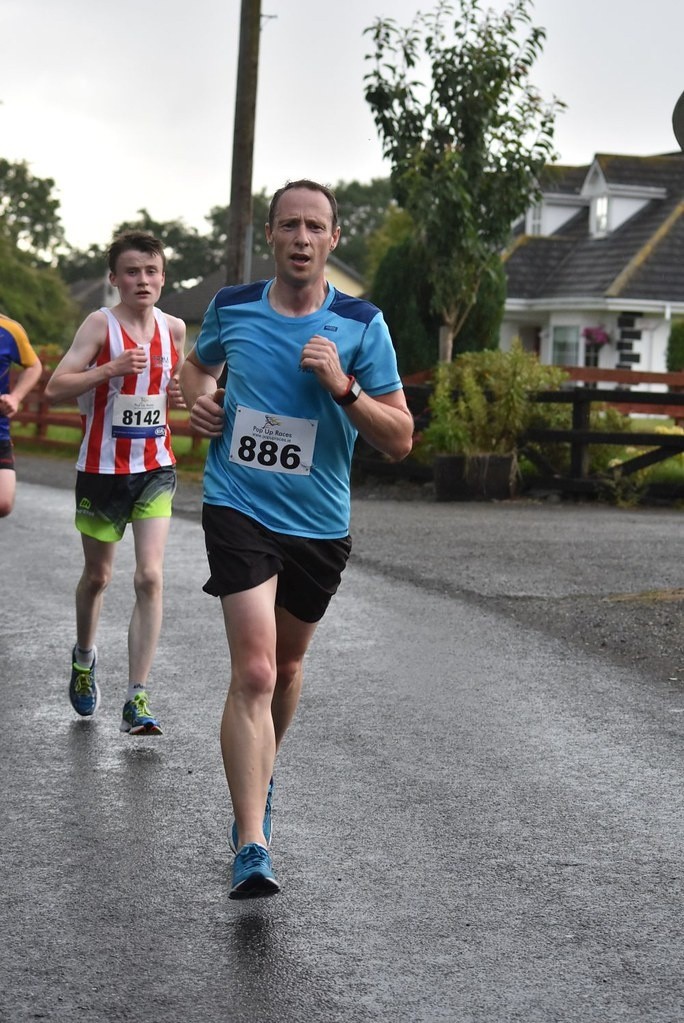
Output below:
[581,327,612,349]
[608,424,684,468]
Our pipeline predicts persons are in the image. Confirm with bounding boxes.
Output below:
[178,178,414,900]
[0,305,43,522]
[45,232,190,740]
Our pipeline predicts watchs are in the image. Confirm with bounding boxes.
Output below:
[330,374,362,408]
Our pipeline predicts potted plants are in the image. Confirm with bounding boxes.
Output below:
[414,333,569,502]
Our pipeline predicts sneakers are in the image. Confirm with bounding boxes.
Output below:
[119,692,164,736]
[230,776,274,855]
[228,841,281,900]
[68,644,100,716]
